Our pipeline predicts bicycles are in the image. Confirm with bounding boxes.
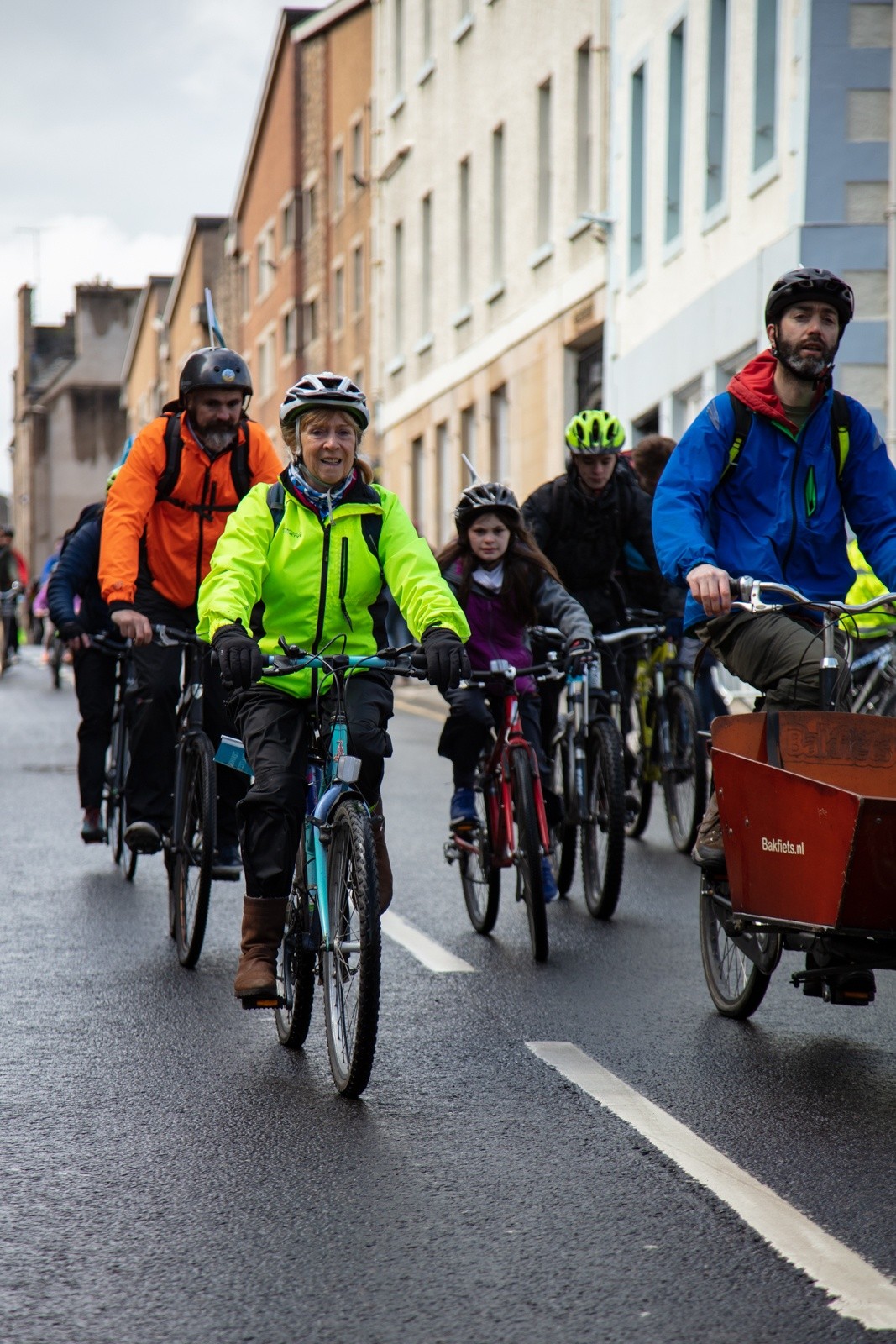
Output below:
[443,575,896,1019]
[0,578,26,668]
[49,622,462,1100]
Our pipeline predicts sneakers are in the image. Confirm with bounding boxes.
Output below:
[449,789,481,832]
[691,790,727,867]
[212,848,242,875]
[806,950,877,993]
[541,856,561,904]
[123,821,161,852]
[81,812,107,842]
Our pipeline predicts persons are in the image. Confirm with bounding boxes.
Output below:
[0,529,28,662]
[652,269,896,1001]
[614,437,699,666]
[432,483,594,902]
[45,465,124,842]
[195,372,471,997]
[99,347,281,872]
[518,409,651,815]
[32,537,81,663]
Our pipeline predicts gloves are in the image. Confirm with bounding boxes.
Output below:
[415,621,472,694]
[564,639,598,678]
[212,617,262,691]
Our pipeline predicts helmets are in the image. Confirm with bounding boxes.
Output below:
[453,482,521,525]
[764,267,854,325]
[564,410,626,456]
[179,346,254,415]
[278,372,373,431]
[104,464,127,496]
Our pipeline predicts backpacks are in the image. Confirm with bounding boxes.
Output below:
[59,503,110,562]
[160,399,257,522]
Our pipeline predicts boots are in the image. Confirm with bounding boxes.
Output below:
[350,794,394,917]
[233,894,289,997]
